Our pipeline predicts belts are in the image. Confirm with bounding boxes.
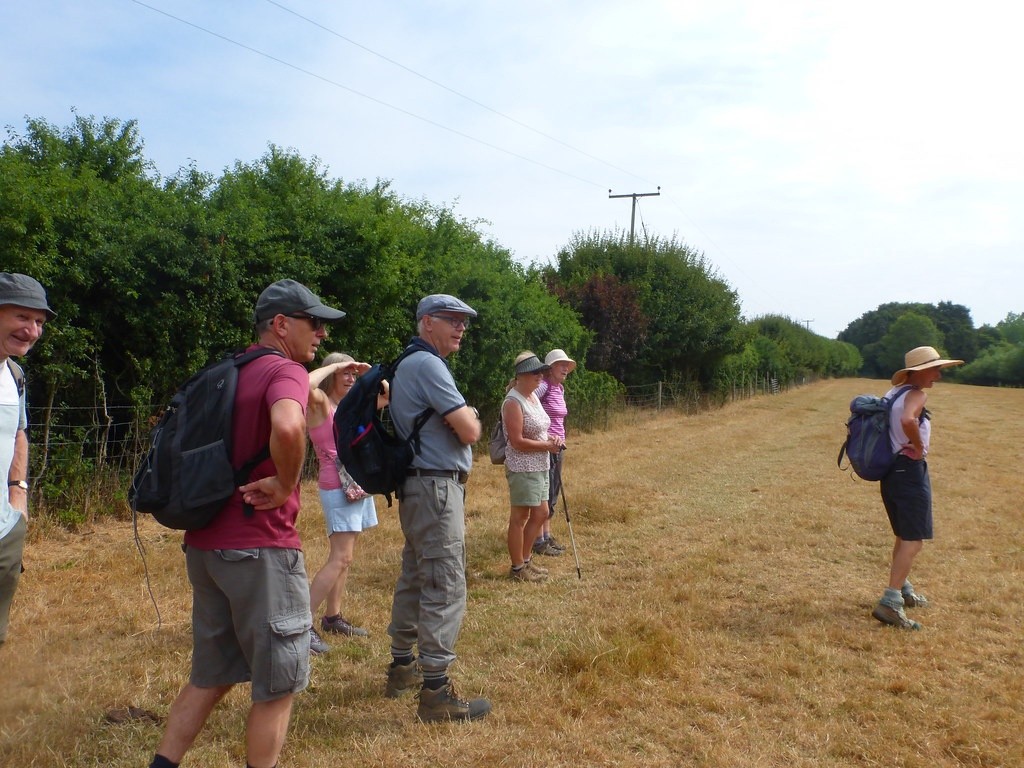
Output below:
[406,468,470,483]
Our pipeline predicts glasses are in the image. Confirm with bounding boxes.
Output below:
[533,369,544,375]
[428,313,469,329]
[269,314,322,331]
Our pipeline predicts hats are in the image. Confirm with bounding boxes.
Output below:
[544,349,576,374]
[515,356,551,374]
[0,273,58,323]
[253,279,347,324]
[892,346,965,385]
[416,294,477,320]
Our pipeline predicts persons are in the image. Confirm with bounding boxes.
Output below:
[500,351,562,581]
[147,277,347,768]
[384,295,492,722]
[533,349,576,557]
[306,353,378,655]
[0,271,61,645]
[871,347,965,630]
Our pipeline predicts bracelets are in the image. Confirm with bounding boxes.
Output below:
[472,407,480,419]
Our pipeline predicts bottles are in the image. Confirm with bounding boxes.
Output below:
[355,426,386,474]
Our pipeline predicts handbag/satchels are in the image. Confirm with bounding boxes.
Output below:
[333,455,369,502]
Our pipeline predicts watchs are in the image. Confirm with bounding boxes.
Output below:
[8,480,28,490]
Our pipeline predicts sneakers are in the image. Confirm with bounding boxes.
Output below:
[872,604,921,632]
[526,555,548,574]
[509,564,547,581]
[545,537,566,550]
[321,611,370,636]
[532,540,562,556]
[309,625,330,655]
[903,593,926,606]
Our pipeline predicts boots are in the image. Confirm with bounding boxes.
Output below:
[414,676,492,722]
[385,655,424,698]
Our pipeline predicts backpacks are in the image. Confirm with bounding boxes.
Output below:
[128,349,287,531]
[332,345,435,494]
[488,395,524,465]
[844,385,925,481]
